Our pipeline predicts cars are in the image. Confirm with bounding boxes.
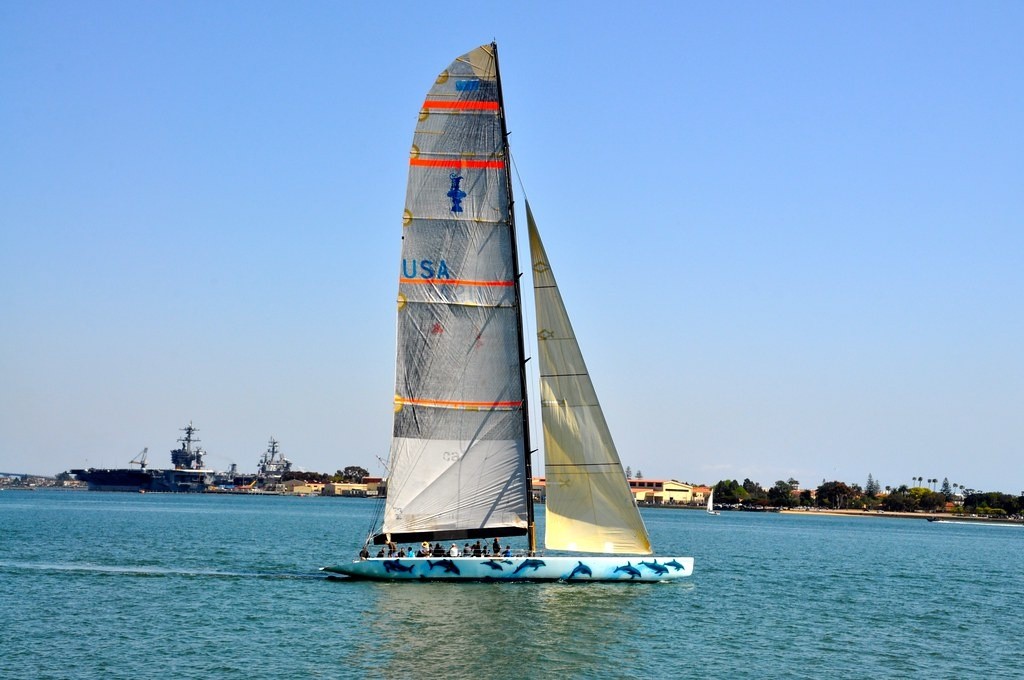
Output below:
[956,513,1024,524]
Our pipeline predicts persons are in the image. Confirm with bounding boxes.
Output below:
[447,543,459,557]
[471,541,481,557]
[385,540,398,557]
[405,547,414,557]
[463,543,472,556]
[481,546,490,557]
[359,546,370,559]
[493,538,500,557]
[376,548,385,557]
[502,546,510,557]
[398,547,406,557]
[433,543,445,556]
[420,542,433,557]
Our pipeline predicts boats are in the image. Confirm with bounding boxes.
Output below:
[67,420,292,495]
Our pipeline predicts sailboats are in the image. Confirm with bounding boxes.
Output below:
[706,488,721,516]
[317,36,695,582]
